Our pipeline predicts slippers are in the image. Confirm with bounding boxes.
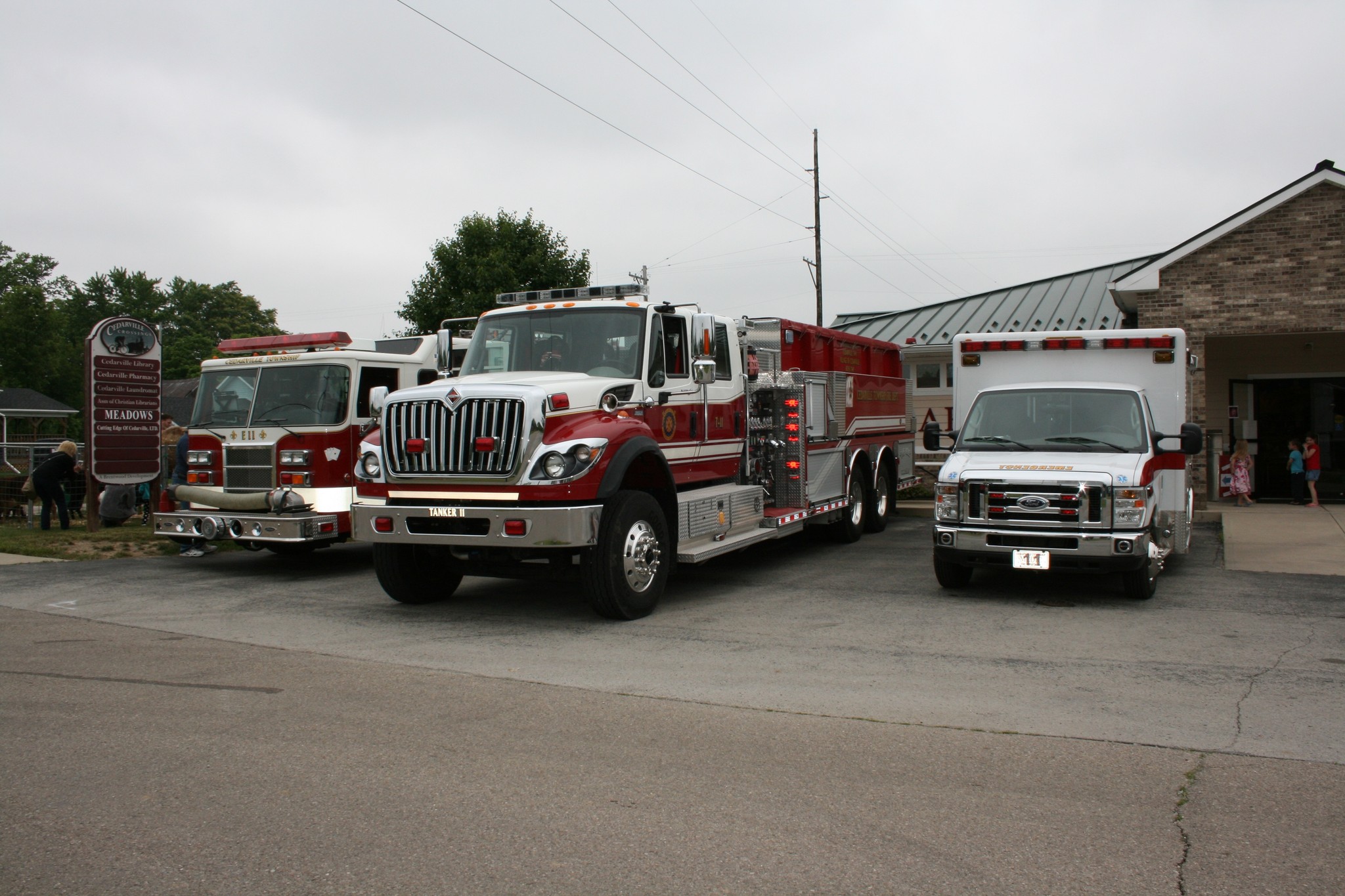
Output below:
[1308,502,1321,507]
[1244,501,1253,507]
[1233,502,1243,507]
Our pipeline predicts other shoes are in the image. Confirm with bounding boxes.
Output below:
[1295,502,1305,505]
[141,523,147,525]
[117,520,124,526]
[1290,501,1295,504]
[158,526,161,529]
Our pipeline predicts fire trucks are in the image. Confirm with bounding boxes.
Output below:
[151,332,508,557]
[920,328,1203,602]
[348,284,919,624]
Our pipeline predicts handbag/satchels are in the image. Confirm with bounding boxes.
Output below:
[21,470,38,500]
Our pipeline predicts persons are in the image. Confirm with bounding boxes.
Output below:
[1301,432,1321,508]
[99,481,138,529]
[1286,439,1304,506]
[139,480,161,528]
[1230,439,1254,507]
[171,425,219,558]
[161,413,180,491]
[31,439,84,530]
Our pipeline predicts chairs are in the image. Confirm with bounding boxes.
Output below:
[627,336,673,374]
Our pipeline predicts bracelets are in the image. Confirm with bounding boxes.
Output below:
[1304,448,1308,451]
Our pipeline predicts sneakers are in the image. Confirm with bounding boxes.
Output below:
[179,547,205,557]
[197,545,218,552]
